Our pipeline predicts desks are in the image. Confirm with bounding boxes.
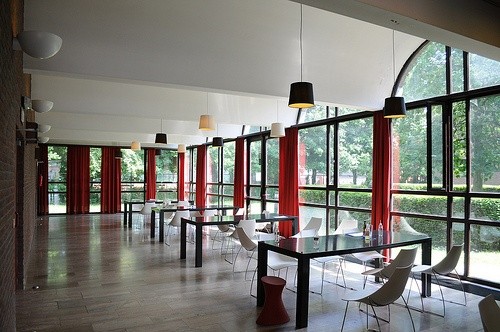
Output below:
[180,213,299,268]
[256,230,432,330]
[150,205,238,243]
[124,200,194,228]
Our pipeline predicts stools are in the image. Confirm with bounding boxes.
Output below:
[256,276,291,326]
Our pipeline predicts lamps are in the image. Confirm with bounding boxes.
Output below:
[130,4,406,153]
[13,30,62,144]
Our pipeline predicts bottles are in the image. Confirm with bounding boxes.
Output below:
[274,224,279,242]
[378,220,383,237]
[362,221,367,238]
[313,230,319,249]
[248,205,251,217]
[365,224,370,239]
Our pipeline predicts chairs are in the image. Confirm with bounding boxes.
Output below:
[135,198,500,332]
